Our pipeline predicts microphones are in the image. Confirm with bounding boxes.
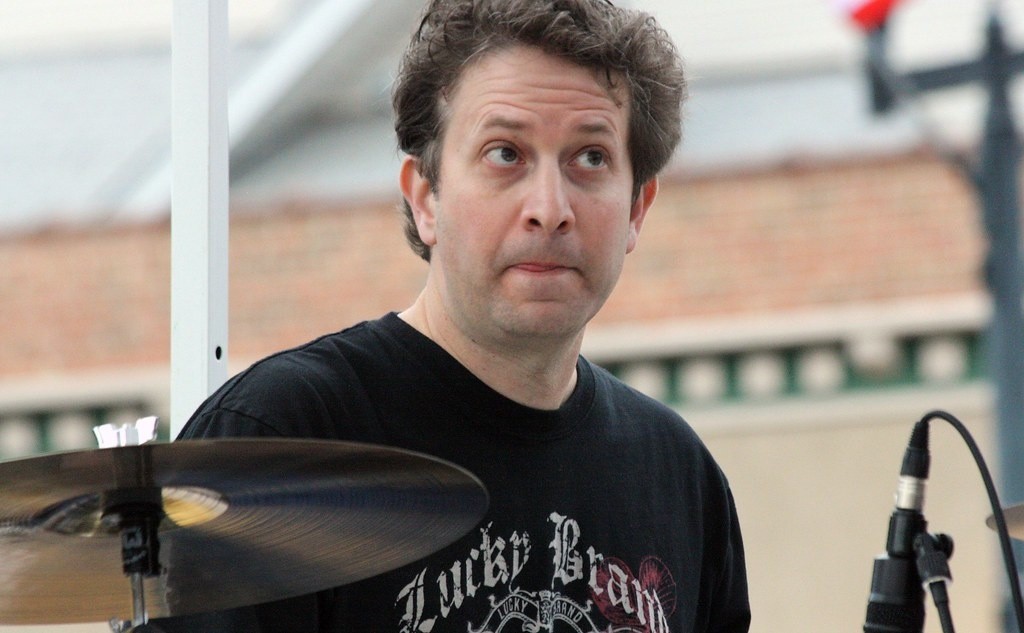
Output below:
[864,419,930,633]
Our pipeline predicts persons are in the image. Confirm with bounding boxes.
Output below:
[135,0,751,633]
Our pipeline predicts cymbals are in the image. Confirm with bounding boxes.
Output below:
[0,438,490,627]
